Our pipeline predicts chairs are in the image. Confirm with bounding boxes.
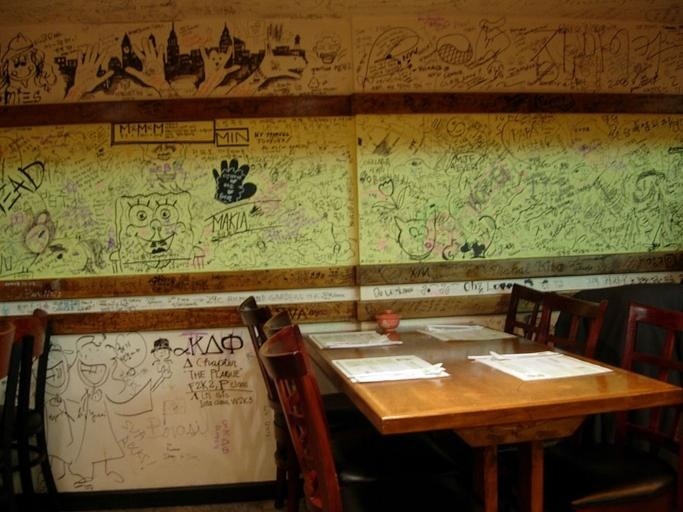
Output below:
[0,307,65,507]
[240,296,466,511]
[504,284,682,509]
